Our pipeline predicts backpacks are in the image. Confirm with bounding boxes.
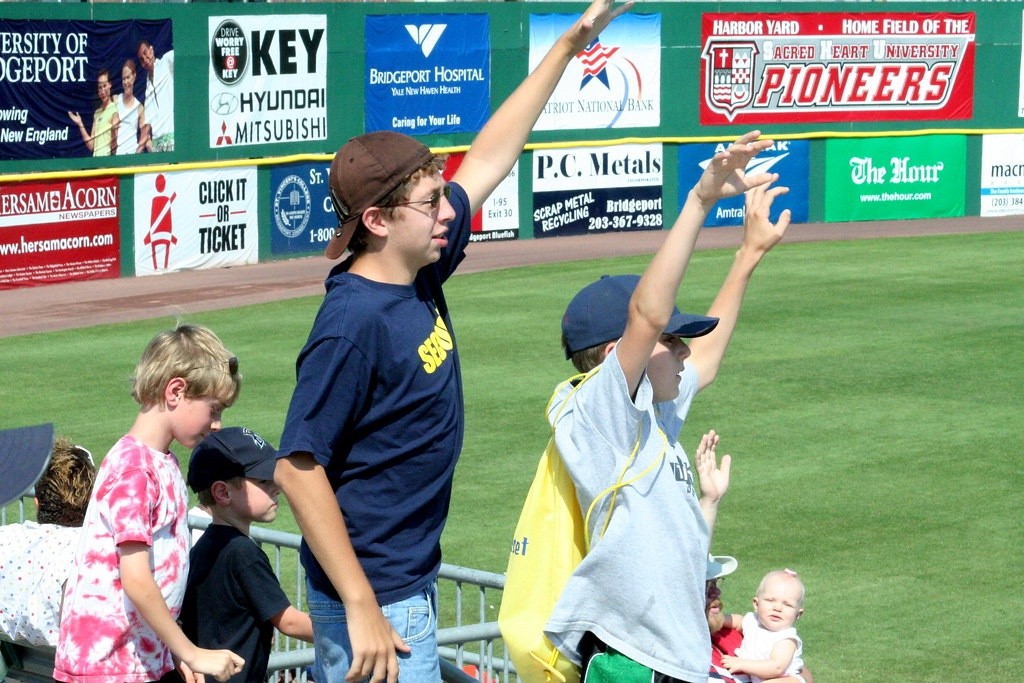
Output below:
[498,361,668,683]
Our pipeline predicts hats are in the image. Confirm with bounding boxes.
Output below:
[561,275,720,361]
[326,130,436,260]
[706,553,738,580]
[187,427,278,494]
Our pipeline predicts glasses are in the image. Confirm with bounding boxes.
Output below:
[75,446,95,467]
[378,185,451,207]
[704,577,726,593]
[181,356,238,375]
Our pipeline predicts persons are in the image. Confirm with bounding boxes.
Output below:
[275,2,630,683]
[0,318,314,683]
[68,40,176,157]
[543,129,813,683]
